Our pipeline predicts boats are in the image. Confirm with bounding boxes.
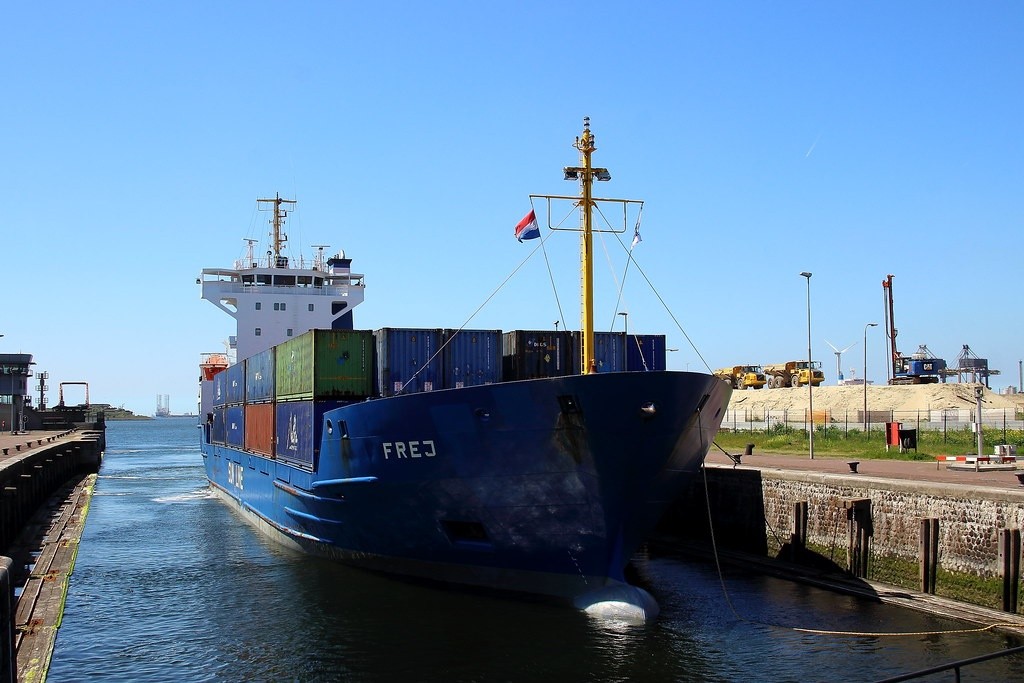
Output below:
[194,116,734,631]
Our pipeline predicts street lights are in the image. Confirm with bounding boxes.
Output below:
[862,323,878,432]
[19,372,26,432]
[800,272,814,460]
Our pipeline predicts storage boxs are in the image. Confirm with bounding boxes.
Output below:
[209,328,667,470]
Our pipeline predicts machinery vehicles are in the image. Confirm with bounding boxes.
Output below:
[764,359,826,389]
[881,274,948,386]
[713,363,767,390]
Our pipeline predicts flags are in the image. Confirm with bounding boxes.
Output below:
[514,209,540,243]
